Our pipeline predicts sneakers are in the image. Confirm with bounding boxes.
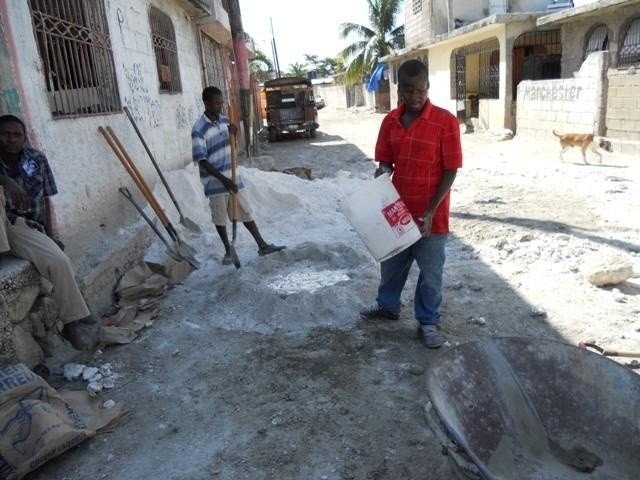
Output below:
[419,320,441,348]
[222,252,233,265]
[359,302,401,320]
[258,244,287,255]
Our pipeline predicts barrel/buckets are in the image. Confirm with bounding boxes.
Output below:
[336,172,423,266]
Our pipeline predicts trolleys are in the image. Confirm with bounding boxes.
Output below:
[419,337,640,480]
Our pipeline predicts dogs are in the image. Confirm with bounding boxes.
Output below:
[551,130,614,167]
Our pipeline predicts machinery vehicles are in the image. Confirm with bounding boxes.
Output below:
[265,75,318,142]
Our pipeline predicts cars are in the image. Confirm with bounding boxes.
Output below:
[314,93,325,109]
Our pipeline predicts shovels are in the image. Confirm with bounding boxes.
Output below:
[96,125,203,270]
[122,105,203,234]
[227,102,242,269]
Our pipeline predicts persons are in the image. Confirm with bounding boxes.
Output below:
[358,58,463,349]
[193,85,287,266]
[0,114,98,350]
